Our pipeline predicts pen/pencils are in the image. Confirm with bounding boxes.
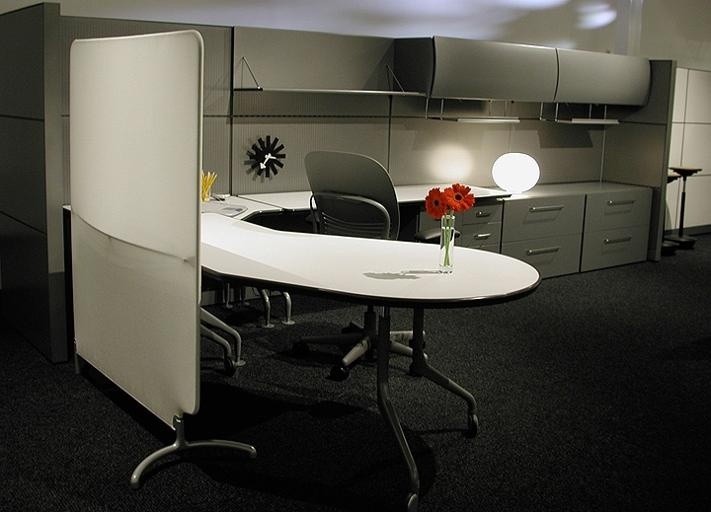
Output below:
[201,170,218,201]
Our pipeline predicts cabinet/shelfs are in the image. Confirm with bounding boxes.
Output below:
[466,182,653,280]
[501,189,539,277]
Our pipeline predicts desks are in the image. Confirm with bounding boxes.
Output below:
[199,195,281,367]
[201,211,540,506]
[236,185,510,325]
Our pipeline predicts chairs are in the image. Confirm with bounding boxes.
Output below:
[304,151,429,381]
